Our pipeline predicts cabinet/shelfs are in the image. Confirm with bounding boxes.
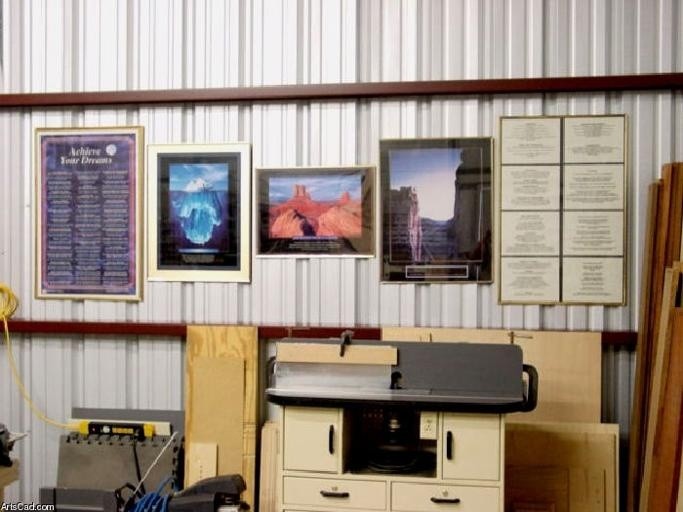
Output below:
[275,406,505,512]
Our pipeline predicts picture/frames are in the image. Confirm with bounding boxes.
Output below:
[35,128,146,301]
[146,142,252,284]
